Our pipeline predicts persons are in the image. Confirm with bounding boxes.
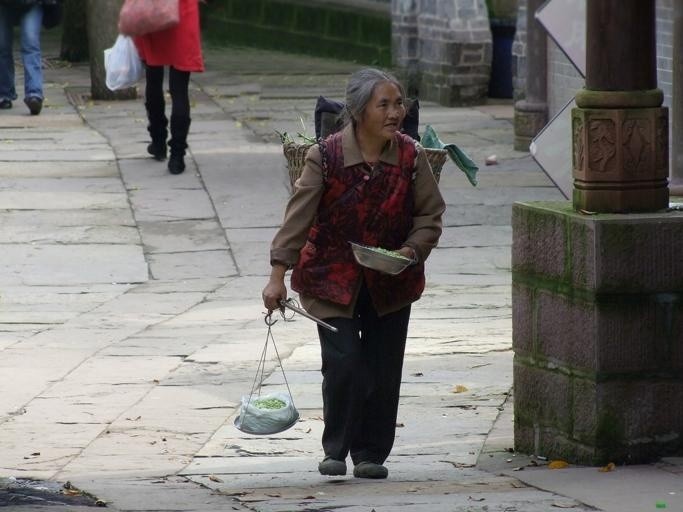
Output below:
[0,0,62,115]
[117,0,204,174]
[262,69,446,477]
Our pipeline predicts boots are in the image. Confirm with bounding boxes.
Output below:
[141,100,169,161]
[167,112,192,174]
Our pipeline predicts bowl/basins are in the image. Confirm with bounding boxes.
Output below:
[347,239,413,276]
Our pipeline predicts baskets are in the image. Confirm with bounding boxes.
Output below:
[280,138,449,207]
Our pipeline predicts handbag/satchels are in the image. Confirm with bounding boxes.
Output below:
[118,0,181,37]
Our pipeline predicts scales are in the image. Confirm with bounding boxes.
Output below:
[233,298,338,435]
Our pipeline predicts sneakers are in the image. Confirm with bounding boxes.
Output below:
[352,459,389,480]
[0,97,12,109]
[317,453,347,476]
[24,95,43,115]
